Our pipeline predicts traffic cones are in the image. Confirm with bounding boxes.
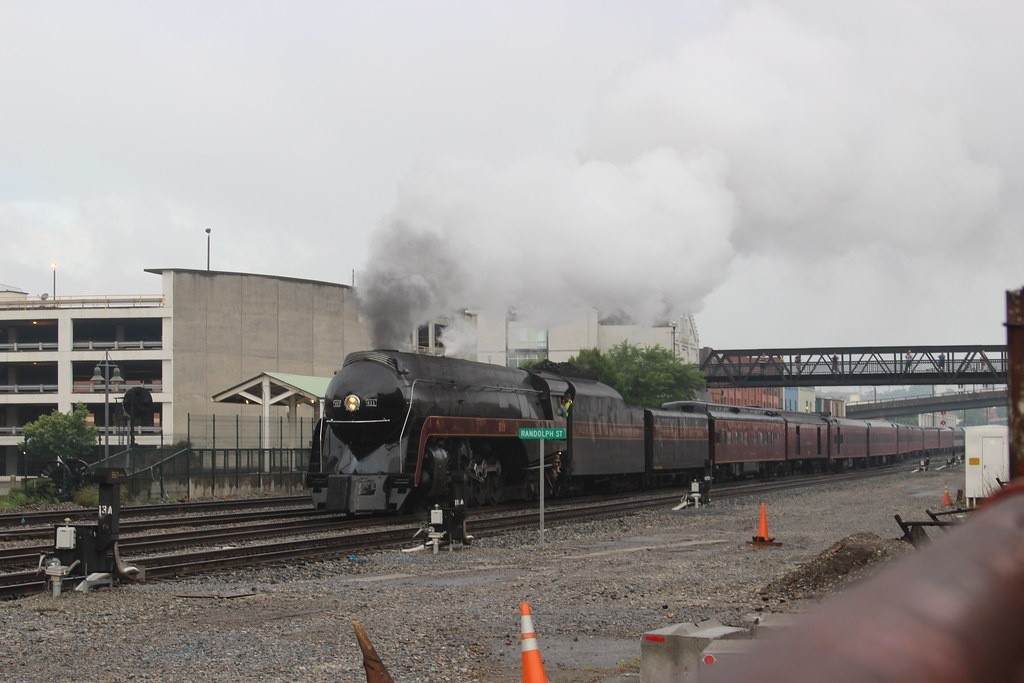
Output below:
[520,601,549,683]
[942,482,952,505]
[755,501,770,541]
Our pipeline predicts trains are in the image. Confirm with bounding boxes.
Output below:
[305,349,964,519]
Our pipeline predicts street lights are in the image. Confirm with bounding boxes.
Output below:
[91,349,126,467]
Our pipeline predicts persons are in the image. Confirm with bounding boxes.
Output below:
[759,353,767,375]
[775,354,785,376]
[795,354,801,375]
[831,354,839,374]
[938,353,945,370]
[904,350,912,373]
